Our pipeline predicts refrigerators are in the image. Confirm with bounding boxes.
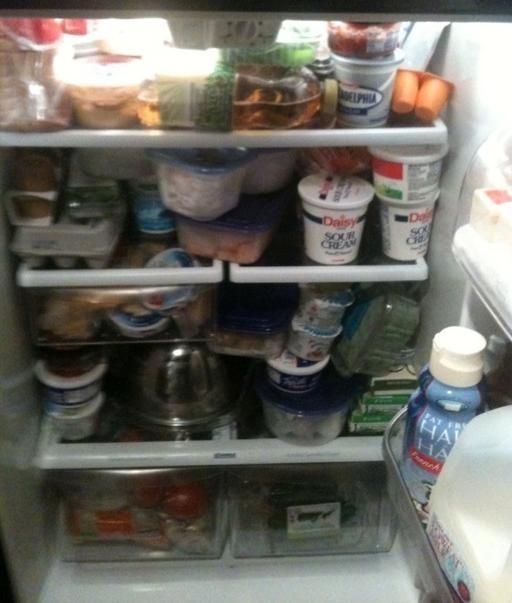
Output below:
[1,0,512,603]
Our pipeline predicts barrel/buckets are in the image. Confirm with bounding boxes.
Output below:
[423,402,512,603]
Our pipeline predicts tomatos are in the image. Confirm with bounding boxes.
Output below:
[127,473,167,507]
[160,479,207,521]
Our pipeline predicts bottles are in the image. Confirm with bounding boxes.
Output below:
[404,323,512,603]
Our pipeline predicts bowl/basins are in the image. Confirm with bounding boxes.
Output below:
[107,343,246,431]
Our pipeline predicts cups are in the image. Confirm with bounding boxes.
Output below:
[150,146,254,224]
[326,39,404,128]
[296,145,446,264]
[28,353,104,438]
[266,283,356,398]
